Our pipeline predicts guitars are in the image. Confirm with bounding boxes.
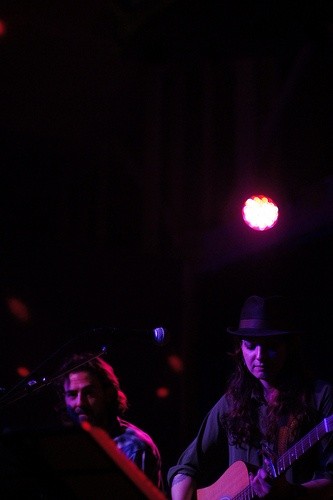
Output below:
[191,413,333,500]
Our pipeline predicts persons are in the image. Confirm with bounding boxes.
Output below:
[55,352,166,499]
[167,295,333,499]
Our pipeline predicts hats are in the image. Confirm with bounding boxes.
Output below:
[226,295,299,337]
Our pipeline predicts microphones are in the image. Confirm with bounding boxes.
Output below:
[91,326,171,347]
